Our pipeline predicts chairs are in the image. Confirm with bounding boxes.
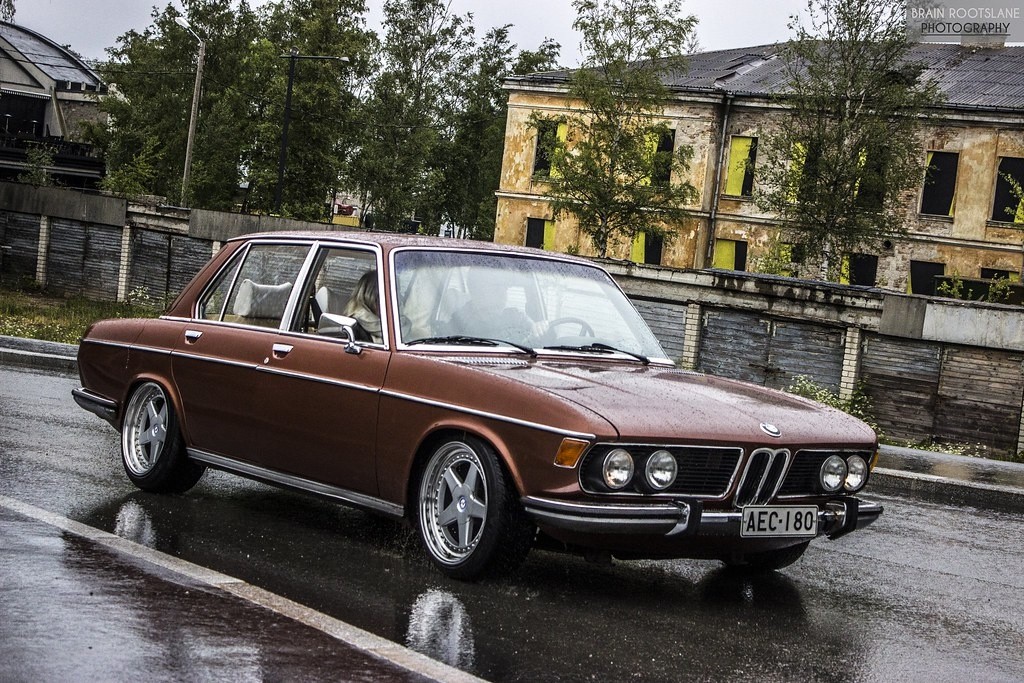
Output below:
[313,287,352,324]
[234,279,293,318]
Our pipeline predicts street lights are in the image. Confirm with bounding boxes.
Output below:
[173,16,206,209]
[272,53,349,213]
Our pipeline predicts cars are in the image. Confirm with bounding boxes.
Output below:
[72,232,885,586]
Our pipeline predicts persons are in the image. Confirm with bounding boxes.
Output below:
[343,268,414,344]
[453,266,551,348]
